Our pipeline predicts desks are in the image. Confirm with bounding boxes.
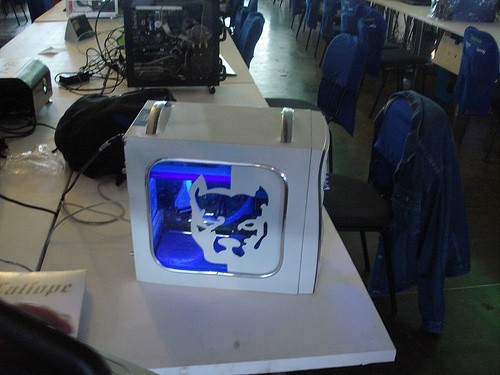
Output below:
[368,0,500,138]
[0,20,255,89]
[0,83,398,375]
[33,0,68,20]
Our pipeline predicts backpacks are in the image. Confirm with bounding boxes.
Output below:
[54,86,177,185]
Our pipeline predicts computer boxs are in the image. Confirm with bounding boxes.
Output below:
[123,0,224,94]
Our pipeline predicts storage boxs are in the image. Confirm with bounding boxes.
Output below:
[119,98,329,295]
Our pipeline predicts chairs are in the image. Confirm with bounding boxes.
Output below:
[9,0,29,26]
[228,0,500,336]
[26,0,55,24]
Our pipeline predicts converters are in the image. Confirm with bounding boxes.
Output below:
[59,70,90,84]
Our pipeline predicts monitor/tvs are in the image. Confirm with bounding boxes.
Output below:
[66,0,118,18]
